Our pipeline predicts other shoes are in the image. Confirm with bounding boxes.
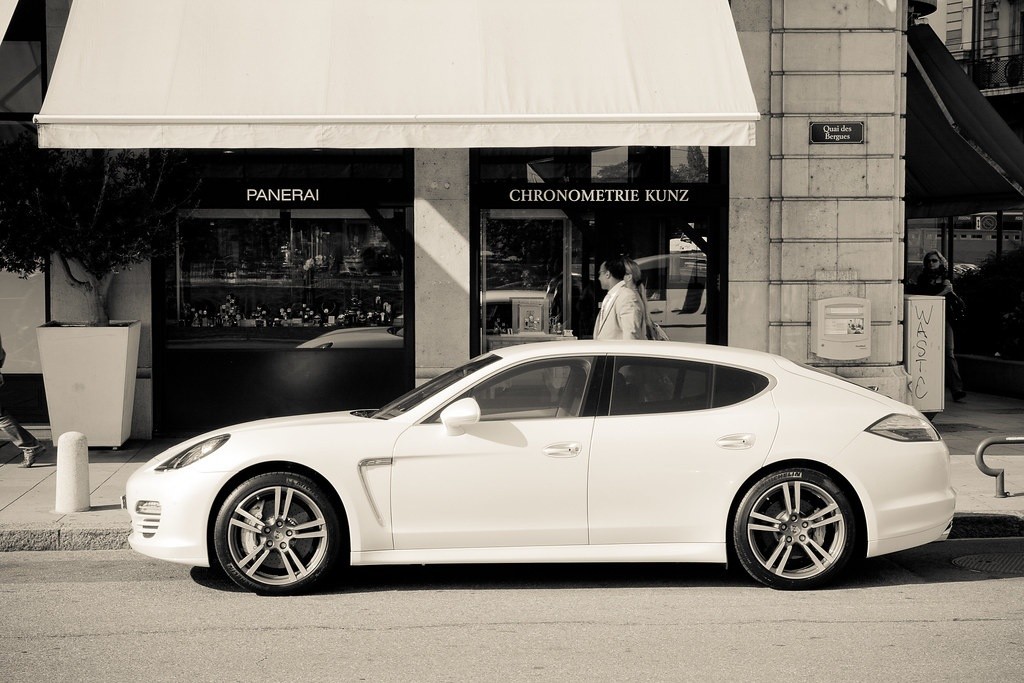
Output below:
[952,390,967,402]
[17,443,45,468]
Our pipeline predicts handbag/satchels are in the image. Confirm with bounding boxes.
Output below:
[940,270,969,324]
[652,320,669,342]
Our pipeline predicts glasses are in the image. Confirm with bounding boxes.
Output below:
[598,270,608,274]
[927,258,941,264]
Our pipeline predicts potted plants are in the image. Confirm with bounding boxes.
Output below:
[0,124,207,450]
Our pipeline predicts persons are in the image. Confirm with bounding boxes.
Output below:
[592,257,673,404]
[0,334,48,468]
[543,257,585,324]
[916,249,967,402]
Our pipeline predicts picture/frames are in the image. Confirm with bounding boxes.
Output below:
[511,297,550,333]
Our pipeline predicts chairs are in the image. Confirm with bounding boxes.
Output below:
[624,366,666,406]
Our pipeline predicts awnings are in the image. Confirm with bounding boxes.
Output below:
[30,1,761,150]
[906,23,1024,220]
[0,0,20,45]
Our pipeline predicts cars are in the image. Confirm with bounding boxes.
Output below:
[119,340,958,595]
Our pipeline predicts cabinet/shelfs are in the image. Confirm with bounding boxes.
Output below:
[484,335,578,354]
